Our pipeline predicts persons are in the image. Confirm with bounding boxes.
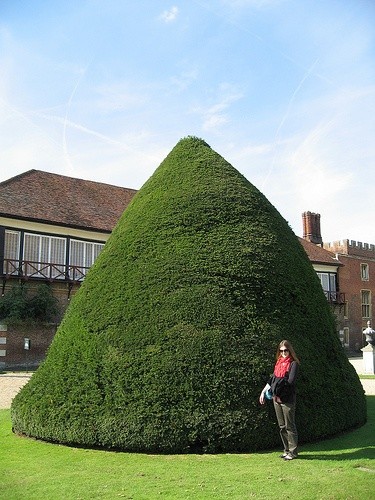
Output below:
[260,339,300,460]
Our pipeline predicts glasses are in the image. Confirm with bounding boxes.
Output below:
[279,349,288,352]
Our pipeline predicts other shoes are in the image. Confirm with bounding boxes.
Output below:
[285,454,297,460]
[280,453,286,457]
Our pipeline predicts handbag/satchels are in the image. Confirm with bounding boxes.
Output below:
[264,385,273,400]
